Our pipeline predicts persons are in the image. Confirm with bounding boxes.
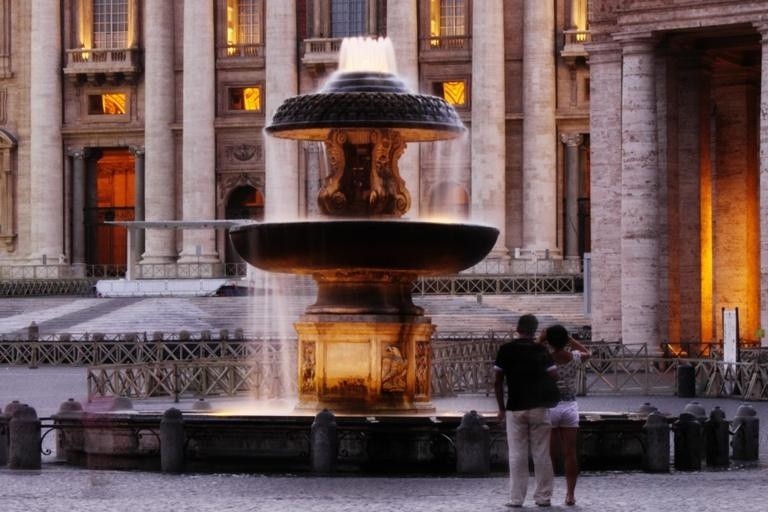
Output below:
[545,323,590,506]
[494,314,559,508]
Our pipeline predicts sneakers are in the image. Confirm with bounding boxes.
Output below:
[504,503,522,507]
[535,499,551,507]
[565,493,575,506]
